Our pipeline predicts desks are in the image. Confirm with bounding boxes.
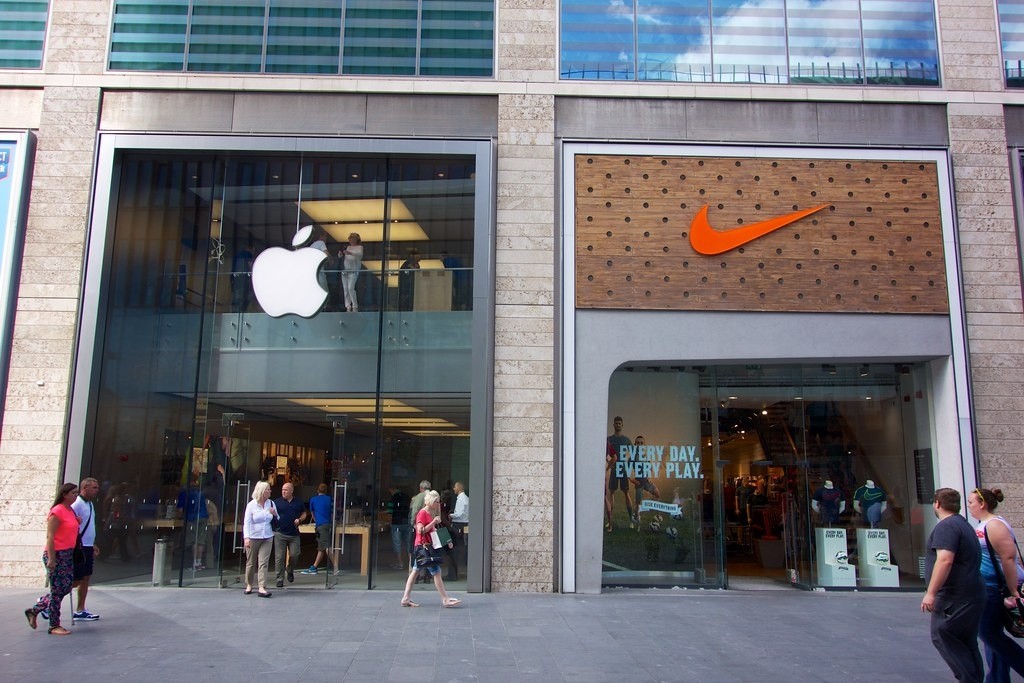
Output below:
[138,516,391,577]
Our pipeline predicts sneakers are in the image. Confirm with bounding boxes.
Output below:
[37,596,49,620]
[605,522,612,532]
[25,609,37,629]
[189,563,206,572]
[392,563,404,570]
[48,626,72,635]
[73,610,100,622]
[326,570,344,576]
[300,566,317,574]
[629,514,640,532]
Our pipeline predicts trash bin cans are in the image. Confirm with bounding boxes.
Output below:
[151,539,175,587]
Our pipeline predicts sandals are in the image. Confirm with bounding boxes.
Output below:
[442,598,461,607]
[401,600,420,608]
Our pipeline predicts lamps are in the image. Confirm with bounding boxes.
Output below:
[274,455,289,487]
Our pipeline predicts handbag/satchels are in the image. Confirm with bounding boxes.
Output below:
[415,526,444,568]
[337,263,343,279]
[73,534,86,566]
[270,515,281,532]
[999,586,1024,638]
[430,527,452,549]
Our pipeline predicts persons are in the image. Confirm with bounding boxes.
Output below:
[360,481,469,607]
[746,489,768,527]
[299,482,342,577]
[97,477,135,567]
[230,243,256,313]
[272,482,306,586]
[176,484,209,572]
[341,233,364,312]
[204,499,218,569]
[440,251,463,311]
[35,479,100,621]
[812,480,846,528]
[629,435,660,531]
[398,251,421,312]
[735,479,753,541]
[852,480,887,529]
[920,487,988,683]
[965,487,1024,683]
[607,415,635,528]
[605,440,617,532]
[242,481,281,597]
[309,232,332,313]
[24,483,82,637]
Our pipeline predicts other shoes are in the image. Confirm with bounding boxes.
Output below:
[347,308,359,312]
[258,591,271,597]
[287,573,294,582]
[441,574,458,581]
[276,581,283,587]
[244,587,252,594]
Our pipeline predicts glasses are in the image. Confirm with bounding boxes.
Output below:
[434,501,443,505]
